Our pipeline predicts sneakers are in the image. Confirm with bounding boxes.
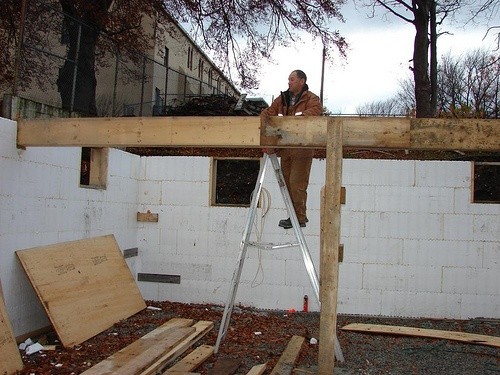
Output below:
[284,217,308,229]
[278,217,306,227]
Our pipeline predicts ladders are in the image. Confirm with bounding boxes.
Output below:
[214,150,343,363]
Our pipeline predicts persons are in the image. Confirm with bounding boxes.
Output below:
[258,70,325,229]
